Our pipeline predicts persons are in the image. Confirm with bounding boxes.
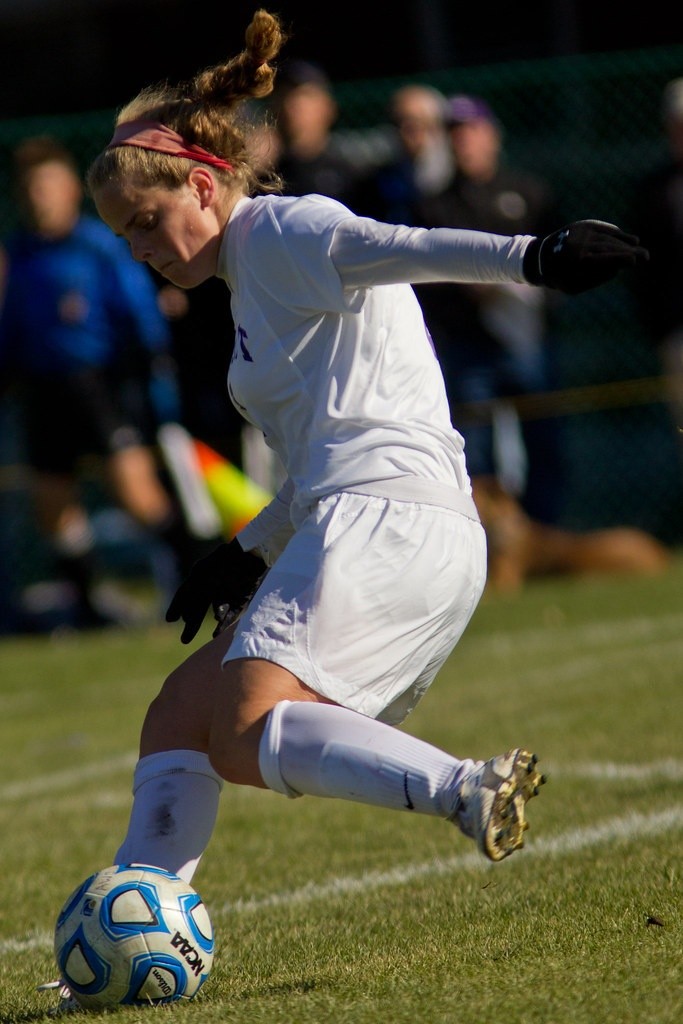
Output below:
[86,6,649,885]
[0,63,682,627]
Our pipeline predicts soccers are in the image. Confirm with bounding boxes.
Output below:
[54,863,215,1010]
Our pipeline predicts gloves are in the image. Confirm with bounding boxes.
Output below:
[166,535,257,645]
[522,215,650,293]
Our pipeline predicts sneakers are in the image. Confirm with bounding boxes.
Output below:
[453,748,547,864]
